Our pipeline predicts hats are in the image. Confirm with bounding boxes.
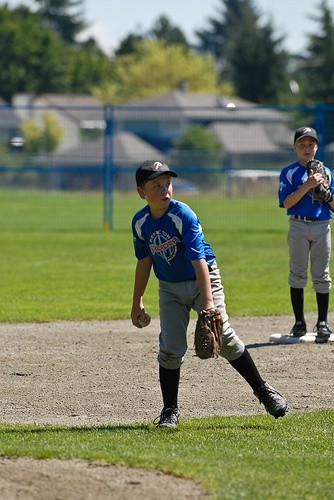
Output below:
[294,126,319,143]
[135,160,177,185]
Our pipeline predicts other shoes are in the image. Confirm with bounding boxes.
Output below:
[312,320,332,338]
[289,321,307,337]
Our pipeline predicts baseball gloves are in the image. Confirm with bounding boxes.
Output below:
[194,307,224,359]
[306,159,333,205]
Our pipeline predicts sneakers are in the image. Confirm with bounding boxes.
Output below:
[153,407,180,427]
[253,381,287,416]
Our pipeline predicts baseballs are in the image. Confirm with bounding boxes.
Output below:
[137,312,151,327]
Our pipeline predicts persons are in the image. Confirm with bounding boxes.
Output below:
[278,126,334,337]
[130,160,287,429]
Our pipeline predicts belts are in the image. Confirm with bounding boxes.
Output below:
[295,215,319,222]
